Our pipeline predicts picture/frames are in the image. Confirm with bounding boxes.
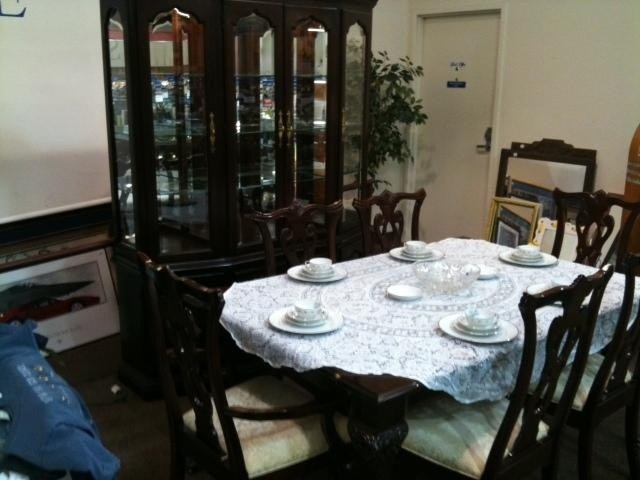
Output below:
[0,240,120,358]
[0,221,116,266]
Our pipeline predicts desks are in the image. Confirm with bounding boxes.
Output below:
[212,236,640,478]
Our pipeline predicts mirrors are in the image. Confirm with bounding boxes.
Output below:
[494,139,597,240]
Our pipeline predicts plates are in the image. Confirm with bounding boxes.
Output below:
[499,251,553,266]
[388,283,422,302]
[527,284,593,305]
[440,312,518,344]
[288,266,347,283]
[389,247,444,262]
[268,307,345,334]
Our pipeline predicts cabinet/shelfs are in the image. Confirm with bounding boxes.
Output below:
[101,0,379,403]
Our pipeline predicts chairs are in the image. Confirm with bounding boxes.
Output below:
[525,250,640,480]
[352,187,427,256]
[255,200,344,275]
[402,261,614,479]
[551,187,640,277]
[157,265,353,479]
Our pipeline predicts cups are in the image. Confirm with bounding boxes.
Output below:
[402,241,427,257]
[414,262,482,296]
[294,298,324,317]
[467,309,499,328]
[513,245,541,258]
[304,258,332,274]
